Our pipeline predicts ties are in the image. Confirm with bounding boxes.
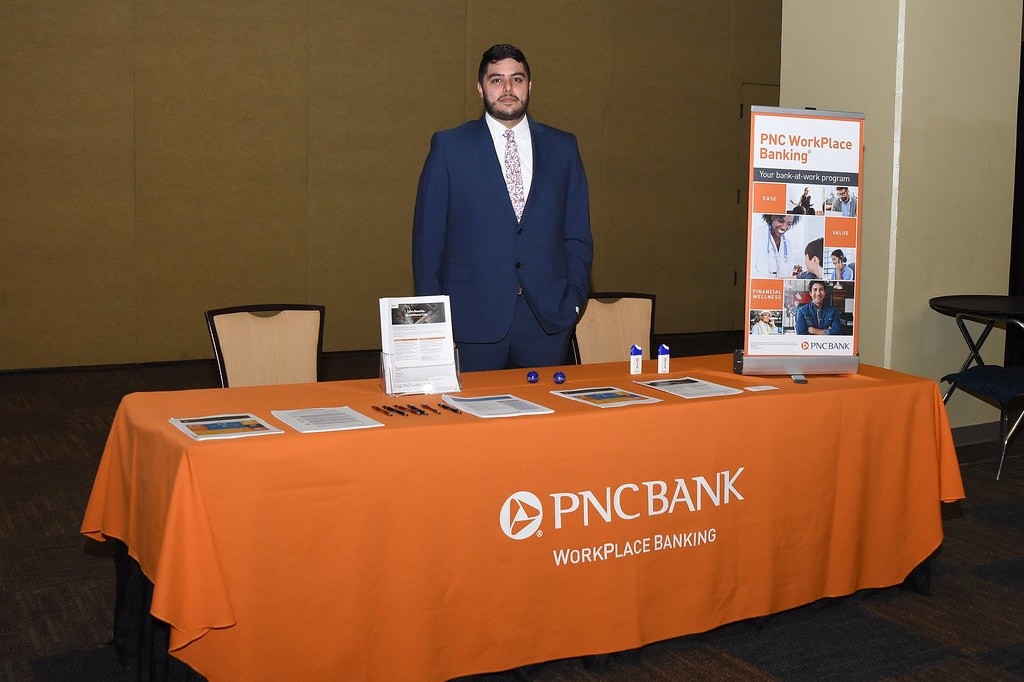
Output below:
[504,129,524,221]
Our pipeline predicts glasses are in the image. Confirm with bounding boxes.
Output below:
[836,190,847,196]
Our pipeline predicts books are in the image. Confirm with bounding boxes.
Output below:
[271,405,385,434]
[441,394,555,419]
[550,387,664,408]
[168,412,284,441]
[631,377,743,399]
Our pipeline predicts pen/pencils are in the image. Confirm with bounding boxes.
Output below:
[382,405,408,417]
[371,405,393,417]
[392,404,420,415]
[406,405,429,415]
[420,403,441,414]
[437,403,462,414]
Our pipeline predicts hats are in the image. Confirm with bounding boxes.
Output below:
[760,310,771,317]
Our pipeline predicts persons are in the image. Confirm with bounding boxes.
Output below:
[798,187,814,210]
[829,250,853,281]
[831,187,856,217]
[781,238,823,280]
[752,217,802,279]
[795,281,840,336]
[412,42,594,375]
[752,311,778,335]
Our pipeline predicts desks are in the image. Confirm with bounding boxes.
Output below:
[79,349,967,682]
[928,294,1023,463]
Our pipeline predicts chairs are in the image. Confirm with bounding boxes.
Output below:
[202,304,325,387]
[927,313,1024,480]
[572,291,655,365]
